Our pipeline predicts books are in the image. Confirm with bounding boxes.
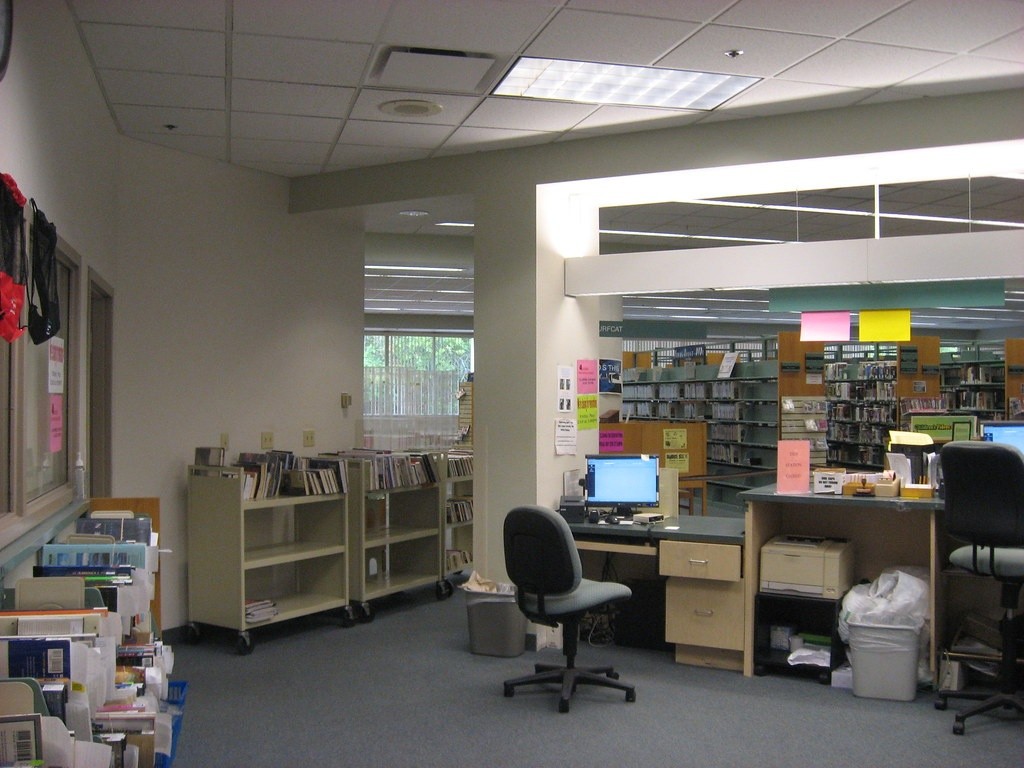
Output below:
[622,362,1005,467]
[1,448,473,768]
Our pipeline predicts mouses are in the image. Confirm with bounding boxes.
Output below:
[605,515,619,525]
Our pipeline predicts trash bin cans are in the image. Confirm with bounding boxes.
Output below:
[847,612,925,701]
[464,583,528,658]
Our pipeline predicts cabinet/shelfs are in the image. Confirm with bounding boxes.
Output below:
[355,453,449,622]
[186,458,359,653]
[441,451,472,598]
[597,322,1022,518]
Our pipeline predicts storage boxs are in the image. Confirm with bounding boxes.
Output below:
[845,613,926,702]
[770,622,799,652]
[155,680,189,768]
[830,661,852,689]
[752,591,841,671]
[812,467,847,496]
[939,660,973,692]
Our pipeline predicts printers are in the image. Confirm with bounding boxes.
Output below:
[760,534,855,600]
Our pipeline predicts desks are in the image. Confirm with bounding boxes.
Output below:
[561,515,747,672]
[736,479,1024,698]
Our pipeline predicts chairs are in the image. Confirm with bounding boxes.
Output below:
[931,435,1024,740]
[504,504,643,718]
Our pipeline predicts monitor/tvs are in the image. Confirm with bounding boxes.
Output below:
[981,420,1024,456]
[586,454,659,517]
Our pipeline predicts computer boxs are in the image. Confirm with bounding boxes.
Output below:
[612,579,671,651]
